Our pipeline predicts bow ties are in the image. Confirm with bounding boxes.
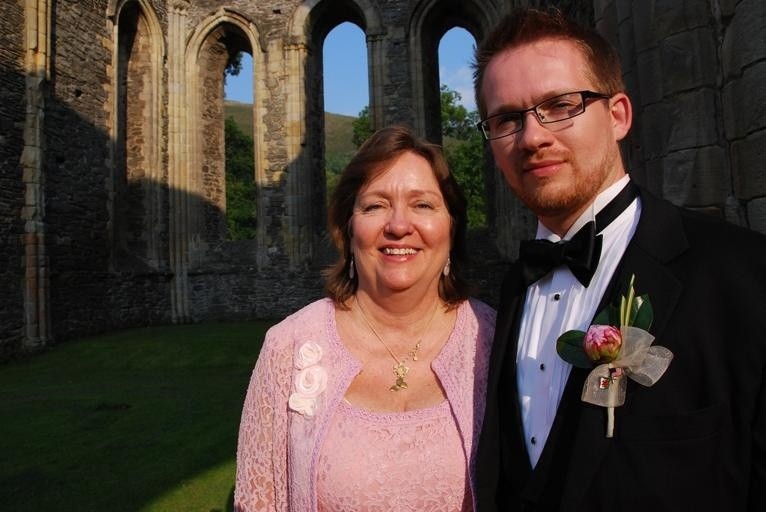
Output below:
[517,220,603,288]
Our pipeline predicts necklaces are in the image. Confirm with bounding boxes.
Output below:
[355,298,438,392]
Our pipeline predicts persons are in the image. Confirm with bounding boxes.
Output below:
[234,125,497,512]
[469,4,766,512]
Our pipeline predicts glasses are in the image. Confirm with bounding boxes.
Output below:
[477,91,612,142]
